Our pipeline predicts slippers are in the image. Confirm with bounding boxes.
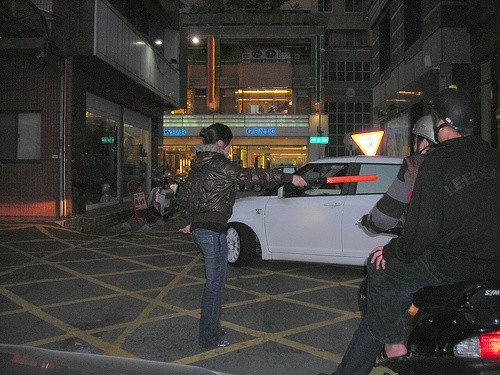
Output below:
[373,349,412,367]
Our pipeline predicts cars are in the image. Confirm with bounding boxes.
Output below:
[251,165,302,193]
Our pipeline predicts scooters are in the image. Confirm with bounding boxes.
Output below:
[146,168,187,225]
[355,213,500,375]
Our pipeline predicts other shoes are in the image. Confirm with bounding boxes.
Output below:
[215,330,230,347]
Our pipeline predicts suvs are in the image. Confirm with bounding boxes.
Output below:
[226,156,407,267]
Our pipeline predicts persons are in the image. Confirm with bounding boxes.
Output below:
[175,123,307,348]
[333,89,500,375]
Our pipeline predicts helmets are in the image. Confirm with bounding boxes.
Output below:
[430,85,481,129]
[411,113,440,145]
